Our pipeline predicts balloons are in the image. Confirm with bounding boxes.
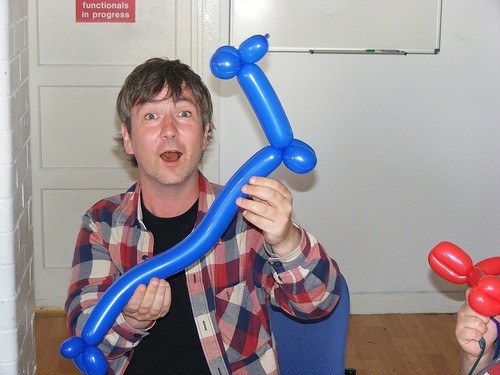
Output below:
[427,242,500,316]
[59,32,316,375]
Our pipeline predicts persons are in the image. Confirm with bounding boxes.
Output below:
[456,287,500,375]
[65,58,339,375]
